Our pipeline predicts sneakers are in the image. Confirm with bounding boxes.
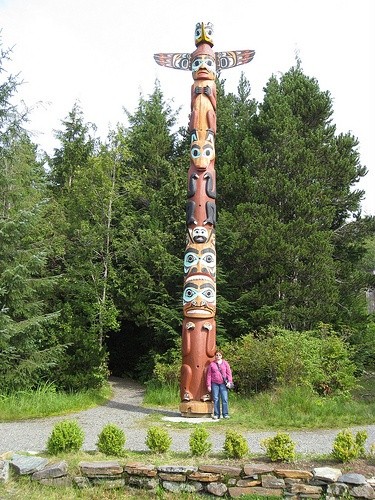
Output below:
[223,415,230,419]
[212,415,219,419]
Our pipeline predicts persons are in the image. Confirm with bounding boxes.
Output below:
[206,350,234,420]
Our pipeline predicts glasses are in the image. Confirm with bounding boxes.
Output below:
[215,354,221,357]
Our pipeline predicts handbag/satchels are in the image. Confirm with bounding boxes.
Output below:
[223,379,234,389]
